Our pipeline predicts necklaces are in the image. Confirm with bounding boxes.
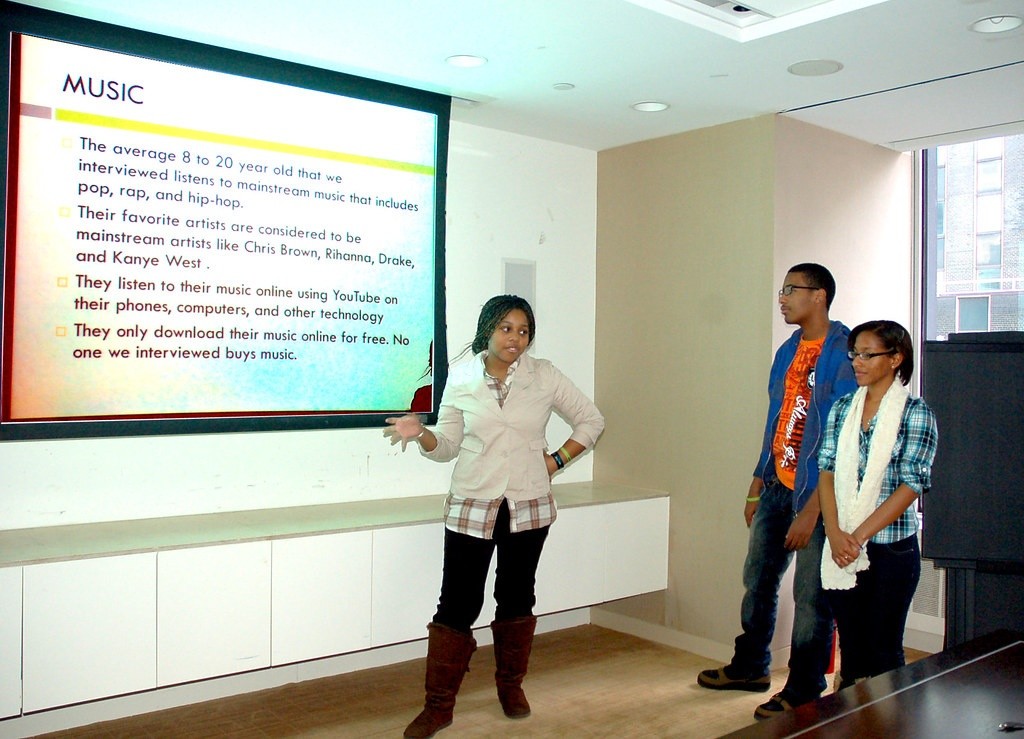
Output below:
[860,411,873,426]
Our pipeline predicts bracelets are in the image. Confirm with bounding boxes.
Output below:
[416,423,426,438]
[560,446,572,461]
[746,496,760,502]
[551,451,565,470]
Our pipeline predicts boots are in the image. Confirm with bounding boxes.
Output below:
[404,622,477,739]
[490,616,537,719]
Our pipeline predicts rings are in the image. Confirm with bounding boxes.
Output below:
[844,555,850,559]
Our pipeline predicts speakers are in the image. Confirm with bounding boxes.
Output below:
[502,257,537,316]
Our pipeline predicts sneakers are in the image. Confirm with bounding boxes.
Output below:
[697,664,770,692]
[753,692,792,720]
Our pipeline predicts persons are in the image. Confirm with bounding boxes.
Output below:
[697,261,859,722]
[382,295,605,739]
[817,321,938,691]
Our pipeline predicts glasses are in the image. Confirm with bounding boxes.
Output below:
[779,284,818,298]
[848,349,898,360]
